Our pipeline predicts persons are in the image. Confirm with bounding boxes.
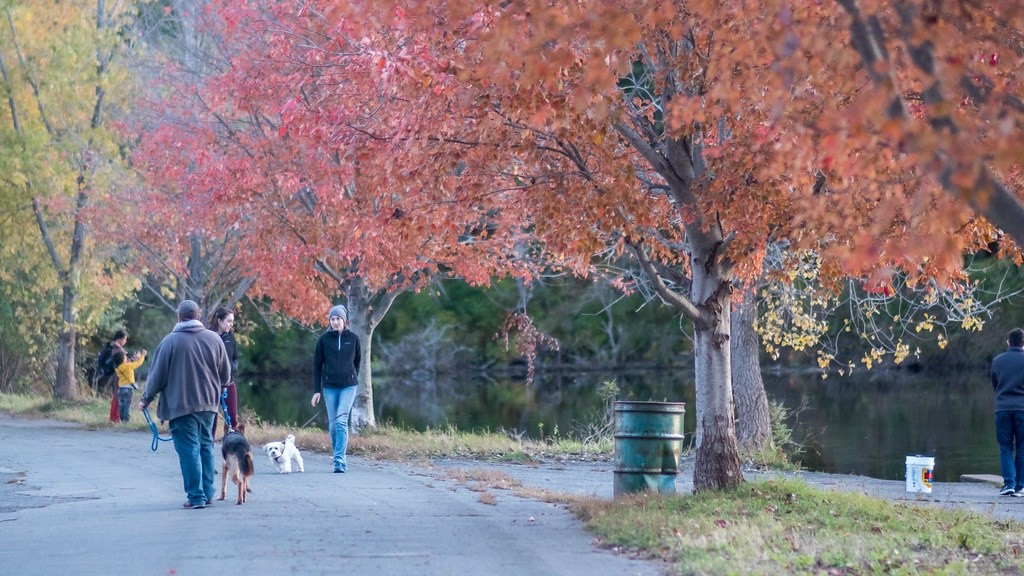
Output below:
[991,328,1024,497]
[311,304,362,473]
[206,307,239,474]
[107,329,147,426]
[138,300,232,508]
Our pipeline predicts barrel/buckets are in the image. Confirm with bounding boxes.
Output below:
[613,401,685,497]
[905,456,935,492]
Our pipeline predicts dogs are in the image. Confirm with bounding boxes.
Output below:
[216,421,255,507]
[262,434,306,473]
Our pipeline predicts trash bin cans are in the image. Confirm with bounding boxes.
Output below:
[612,400,687,501]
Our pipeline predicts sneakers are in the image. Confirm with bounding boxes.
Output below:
[334,463,346,472]
[182,500,205,508]
[999,485,1015,495]
[1011,488,1024,497]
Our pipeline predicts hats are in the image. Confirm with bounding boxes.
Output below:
[329,304,347,324]
[176,300,199,317]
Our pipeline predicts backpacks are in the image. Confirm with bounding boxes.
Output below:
[98,342,115,377]
